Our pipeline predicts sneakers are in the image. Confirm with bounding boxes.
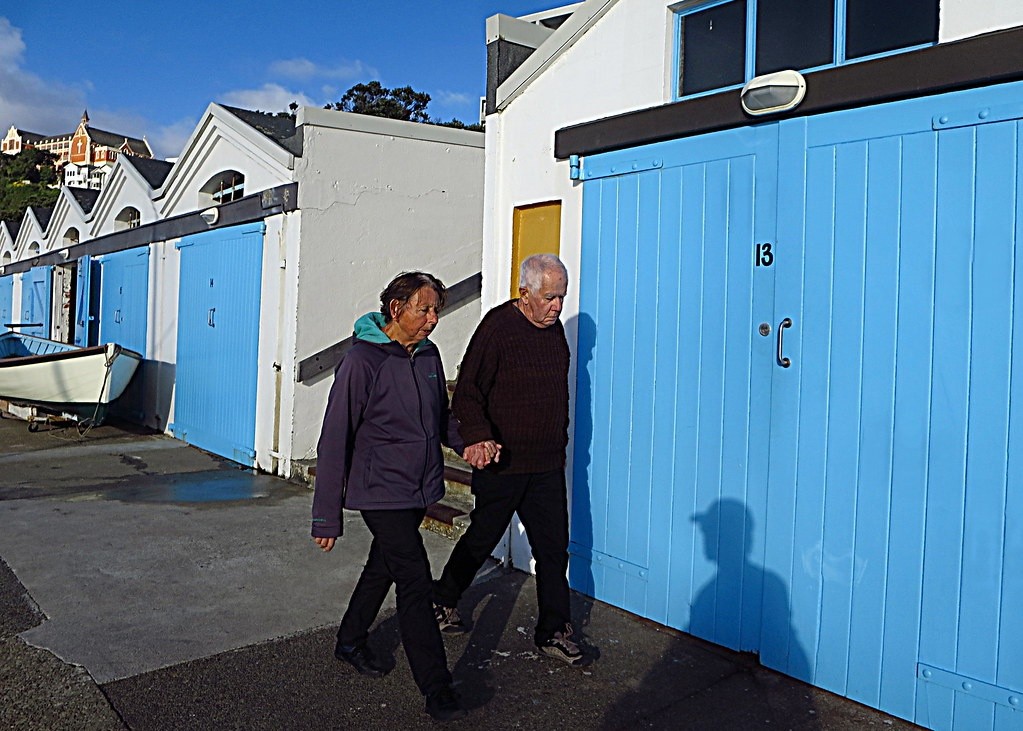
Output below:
[334,641,388,678]
[432,602,465,636]
[536,622,601,670]
[424,687,467,721]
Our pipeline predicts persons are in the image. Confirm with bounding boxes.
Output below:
[433,253,595,669]
[311,271,502,721]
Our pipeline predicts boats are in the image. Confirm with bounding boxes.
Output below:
[0,331,142,425]
